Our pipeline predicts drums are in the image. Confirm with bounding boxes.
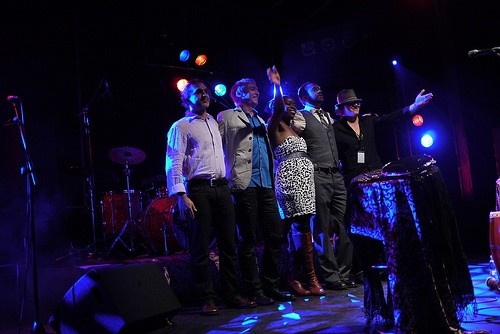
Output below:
[489,211,500,274]
[98,188,145,236]
[138,185,220,251]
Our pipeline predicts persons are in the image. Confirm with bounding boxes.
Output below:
[217,77,296,305]
[267,65,329,298]
[331,89,434,285]
[166,81,258,313]
[290,82,379,290]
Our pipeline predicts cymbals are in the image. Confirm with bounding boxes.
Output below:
[108,145,146,166]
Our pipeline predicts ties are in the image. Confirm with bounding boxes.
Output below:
[315,109,327,126]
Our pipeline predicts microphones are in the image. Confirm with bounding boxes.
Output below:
[6,95,20,101]
[105,80,112,96]
[468,47,500,57]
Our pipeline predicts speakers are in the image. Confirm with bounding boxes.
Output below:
[48,263,183,334]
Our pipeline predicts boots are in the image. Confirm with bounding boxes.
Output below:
[281,236,310,295]
[299,231,326,295]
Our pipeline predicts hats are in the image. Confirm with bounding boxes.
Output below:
[335,88,362,106]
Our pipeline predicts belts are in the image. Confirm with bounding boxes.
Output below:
[314,167,339,171]
[188,178,228,187]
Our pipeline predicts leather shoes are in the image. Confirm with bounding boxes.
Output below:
[250,287,274,305]
[203,299,221,315]
[268,286,294,301]
[345,280,356,288]
[223,294,255,309]
[327,280,347,291]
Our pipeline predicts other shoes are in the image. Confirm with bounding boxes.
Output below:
[355,276,365,285]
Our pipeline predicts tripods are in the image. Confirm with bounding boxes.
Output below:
[104,153,147,261]
